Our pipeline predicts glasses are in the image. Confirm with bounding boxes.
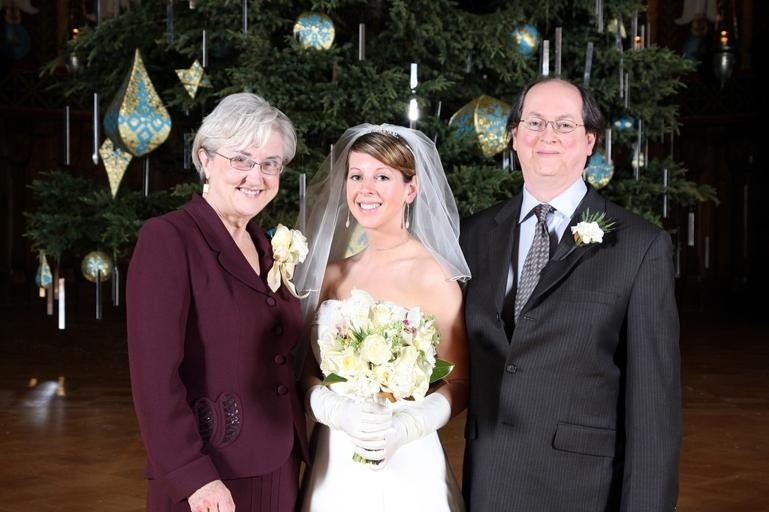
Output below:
[519,116,583,134]
[214,151,283,176]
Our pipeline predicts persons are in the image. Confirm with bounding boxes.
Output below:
[450,76,683,512]
[293,121,471,512]
[124,92,313,512]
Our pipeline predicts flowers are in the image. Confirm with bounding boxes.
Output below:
[316,302,456,464]
[569,206,618,246]
[264,221,313,299]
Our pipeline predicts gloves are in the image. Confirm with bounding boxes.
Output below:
[353,392,452,461]
[310,384,394,442]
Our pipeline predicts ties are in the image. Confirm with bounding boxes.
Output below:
[514,205,555,326]
